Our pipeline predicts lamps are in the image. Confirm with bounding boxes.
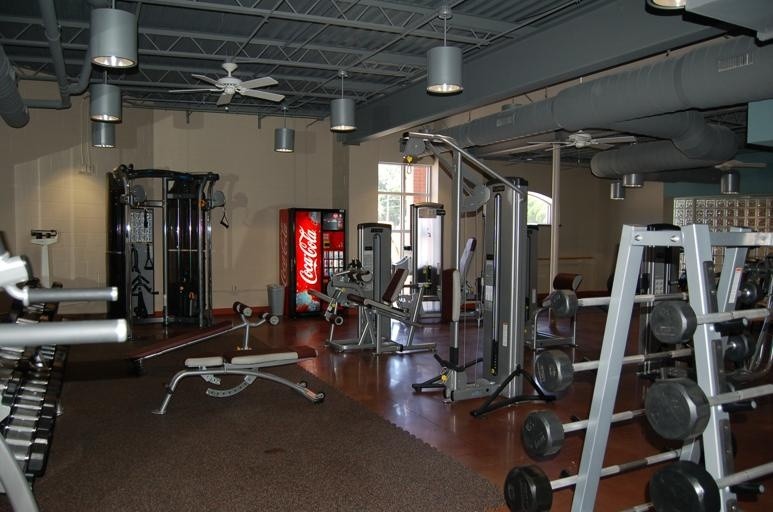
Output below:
[721,169,742,196]
[646,0,690,12]
[89,1,138,71]
[273,105,296,154]
[327,68,357,134]
[426,6,464,94]
[86,69,124,149]
[609,172,644,200]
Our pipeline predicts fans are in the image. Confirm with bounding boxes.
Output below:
[169,62,284,107]
[715,160,765,172]
[529,130,637,153]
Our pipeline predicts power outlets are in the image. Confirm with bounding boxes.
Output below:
[79,164,94,174]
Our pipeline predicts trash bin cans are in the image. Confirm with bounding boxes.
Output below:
[267,284,285,315]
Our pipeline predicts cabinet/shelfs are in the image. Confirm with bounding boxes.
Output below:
[672,194,772,280]
[279,207,348,318]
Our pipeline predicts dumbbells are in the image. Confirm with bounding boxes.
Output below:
[0,309,70,478]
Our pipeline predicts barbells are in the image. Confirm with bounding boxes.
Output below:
[503,282,773,511]
